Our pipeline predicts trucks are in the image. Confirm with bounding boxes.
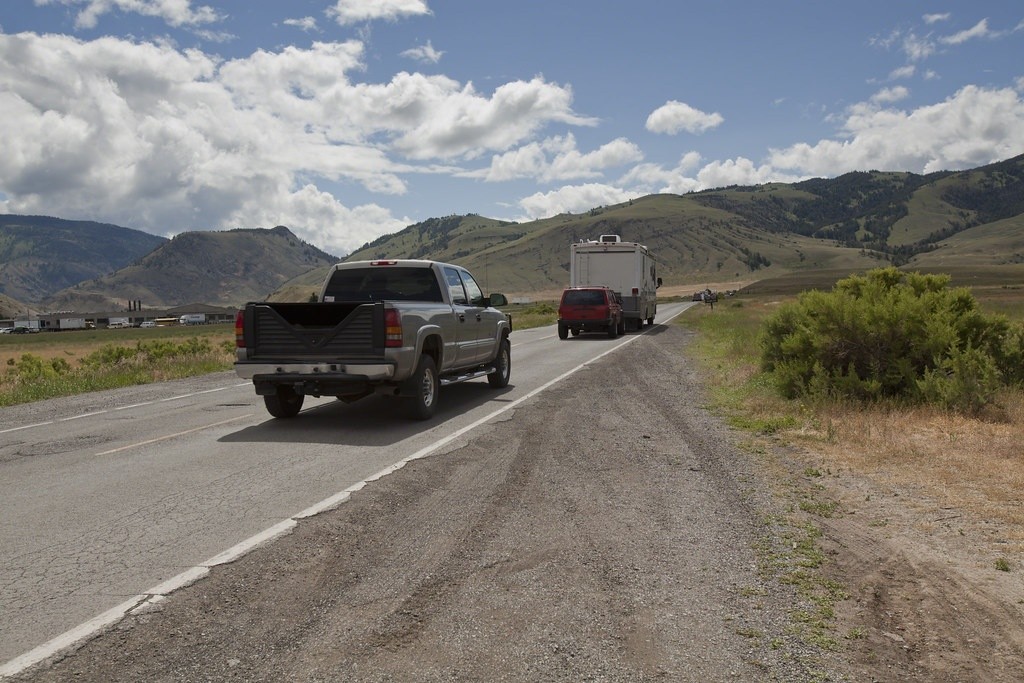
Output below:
[568,234,662,330]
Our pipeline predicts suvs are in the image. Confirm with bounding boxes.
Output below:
[557,285,625,339]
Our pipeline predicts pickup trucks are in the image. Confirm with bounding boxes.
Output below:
[231,258,514,423]
